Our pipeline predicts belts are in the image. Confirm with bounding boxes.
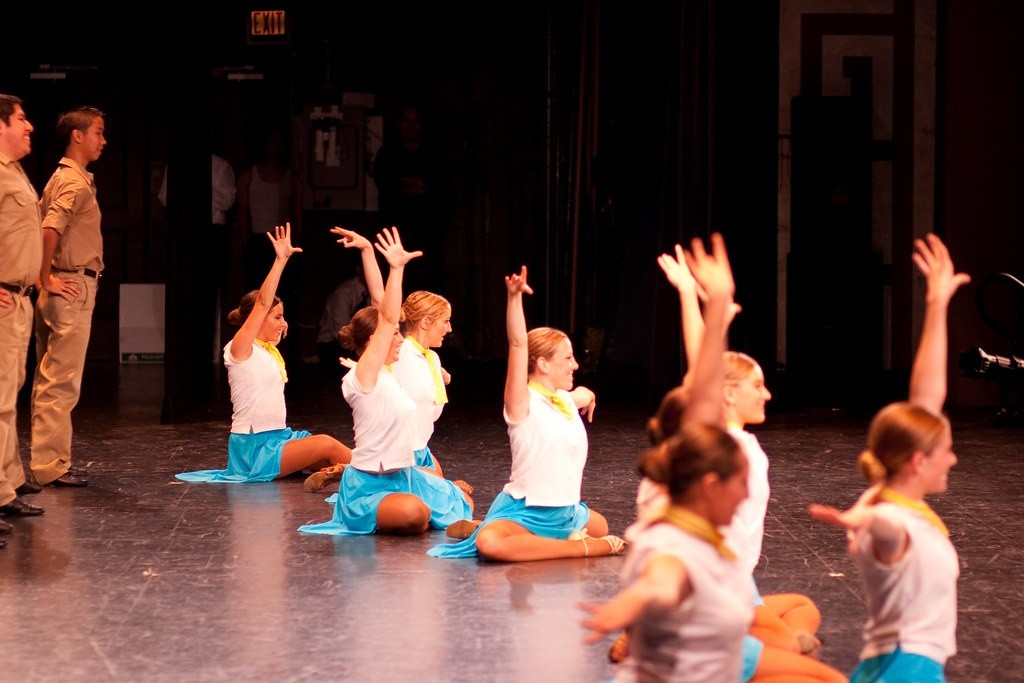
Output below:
[56,263,96,279]
[0,280,33,296]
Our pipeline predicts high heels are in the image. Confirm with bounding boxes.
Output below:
[446,517,483,540]
[304,462,345,492]
[568,526,629,557]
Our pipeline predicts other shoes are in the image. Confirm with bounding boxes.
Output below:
[452,478,474,496]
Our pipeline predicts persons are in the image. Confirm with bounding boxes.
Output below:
[160,102,450,385]
[296,226,474,536]
[174,221,351,483]
[813,232,972,683]
[425,266,626,561]
[577,233,849,683]
[1,94,105,549]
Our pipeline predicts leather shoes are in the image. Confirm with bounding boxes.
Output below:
[67,464,89,476]
[0,519,15,534]
[15,481,41,494]
[49,474,88,488]
[0,497,46,516]
[0,538,8,549]
